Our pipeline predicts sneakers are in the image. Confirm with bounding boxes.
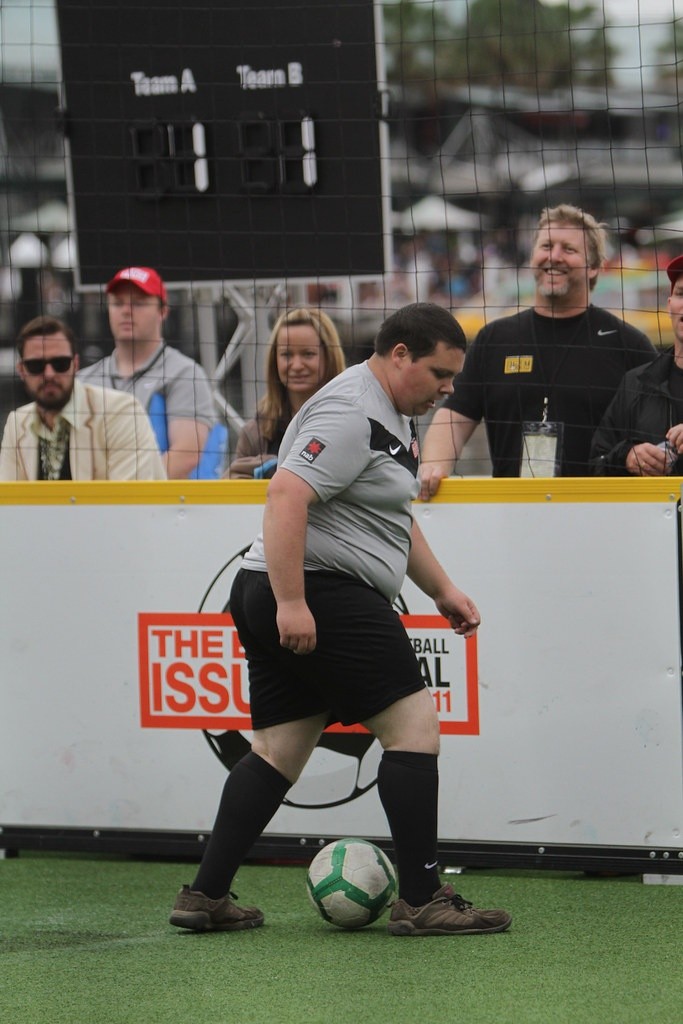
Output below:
[389,878,512,938]
[169,884,264,930]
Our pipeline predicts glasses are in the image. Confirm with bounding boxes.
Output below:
[22,355,75,374]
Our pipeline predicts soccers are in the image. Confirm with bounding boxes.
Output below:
[303,837,398,930]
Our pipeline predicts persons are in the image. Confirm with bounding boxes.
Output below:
[0,313,167,483]
[219,304,347,484]
[73,265,215,478]
[415,203,683,503]
[170,304,513,936]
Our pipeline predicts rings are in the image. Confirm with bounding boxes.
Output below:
[641,461,645,468]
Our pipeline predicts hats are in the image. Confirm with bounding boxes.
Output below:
[666,255,683,296]
[105,267,166,305]
